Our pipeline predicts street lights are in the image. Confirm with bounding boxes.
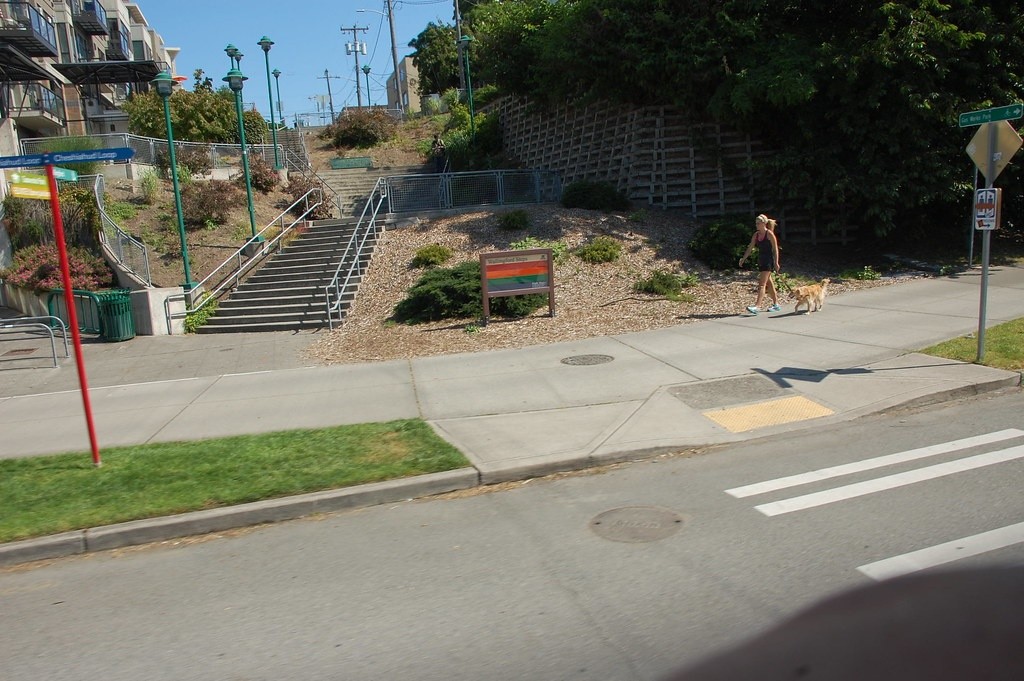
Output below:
[222,44,264,243]
[149,68,197,290]
[257,35,283,169]
[356,9,405,114]
[271,67,282,125]
[458,35,476,146]
[361,64,371,110]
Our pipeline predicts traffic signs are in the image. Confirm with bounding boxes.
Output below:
[958,103,1023,128]
[0,145,140,170]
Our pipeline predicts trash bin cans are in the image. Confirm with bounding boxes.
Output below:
[95,287,136,343]
[293,120,304,127]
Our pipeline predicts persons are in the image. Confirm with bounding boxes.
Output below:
[432,134,445,153]
[739,214,781,313]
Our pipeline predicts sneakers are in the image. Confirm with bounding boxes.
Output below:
[768,304,780,312]
[747,306,759,314]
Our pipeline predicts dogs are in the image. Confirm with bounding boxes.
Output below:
[788,279,830,315]
